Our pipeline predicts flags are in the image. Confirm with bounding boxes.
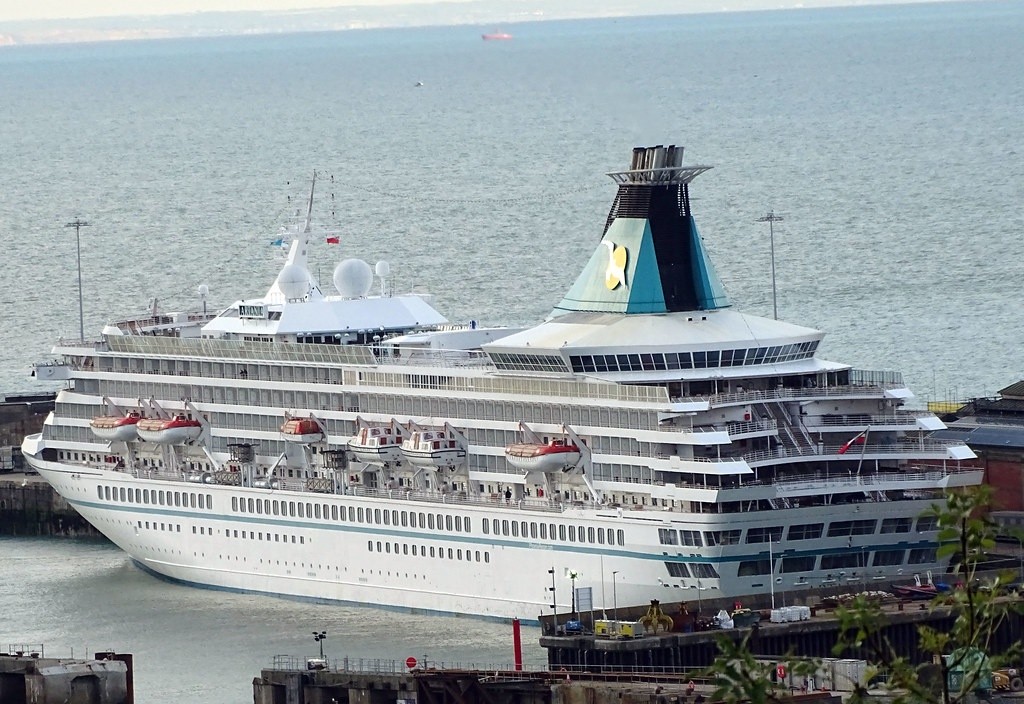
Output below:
[270,239,283,246]
[838,426,869,454]
[327,236,339,244]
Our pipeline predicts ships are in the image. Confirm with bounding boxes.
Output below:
[19,144,987,634]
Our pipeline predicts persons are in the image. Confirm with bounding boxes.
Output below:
[239,368,248,379]
[149,464,158,480]
[180,461,187,478]
[133,458,141,478]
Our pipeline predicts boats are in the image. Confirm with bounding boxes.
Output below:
[504,443,580,473]
[135,415,201,444]
[348,426,403,461]
[398,429,467,473]
[89,414,144,448]
[279,417,323,444]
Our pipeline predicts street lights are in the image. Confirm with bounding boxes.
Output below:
[612,570,620,637]
